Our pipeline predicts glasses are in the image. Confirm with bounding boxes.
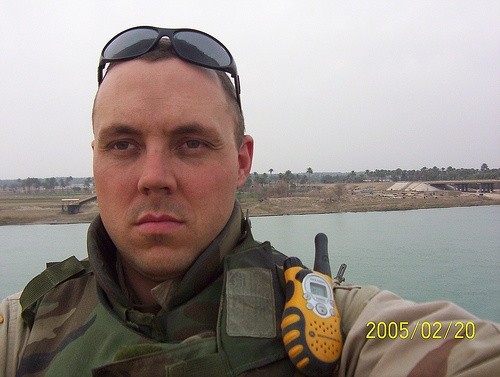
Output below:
[97,25,242,113]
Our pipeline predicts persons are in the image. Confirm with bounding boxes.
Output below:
[0,26,500,377]
